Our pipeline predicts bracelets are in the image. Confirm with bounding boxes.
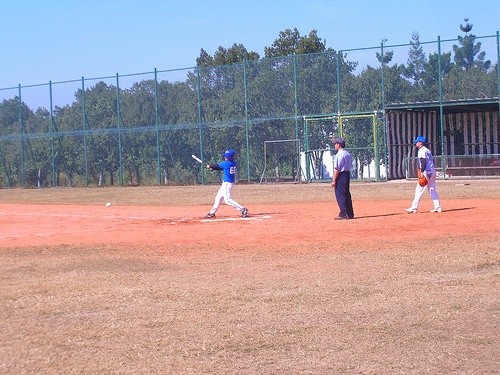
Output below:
[332,181,335,182]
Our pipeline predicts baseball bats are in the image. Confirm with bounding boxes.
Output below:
[191,154,213,171]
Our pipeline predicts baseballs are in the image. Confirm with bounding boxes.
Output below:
[105,201,112,207]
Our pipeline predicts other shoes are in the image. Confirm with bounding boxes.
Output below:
[333,216,349,220]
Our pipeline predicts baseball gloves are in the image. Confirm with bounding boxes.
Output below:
[417,173,429,187]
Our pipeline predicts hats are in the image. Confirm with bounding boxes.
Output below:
[413,136,426,143]
[223,150,236,159]
[332,138,345,145]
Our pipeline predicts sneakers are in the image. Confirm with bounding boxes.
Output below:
[405,208,418,213]
[203,213,216,219]
[430,208,441,212]
[240,208,248,218]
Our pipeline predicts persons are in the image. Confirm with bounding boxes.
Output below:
[331,138,355,220]
[406,136,442,214]
[204,151,249,218]
